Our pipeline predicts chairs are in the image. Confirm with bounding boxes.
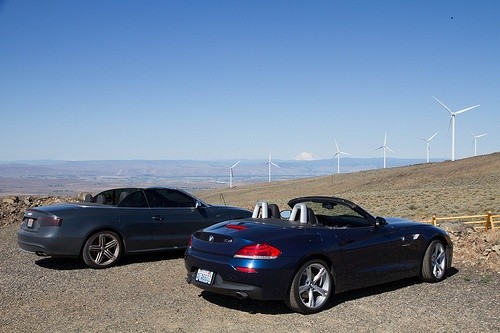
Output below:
[82,191,132,205]
[251,201,319,225]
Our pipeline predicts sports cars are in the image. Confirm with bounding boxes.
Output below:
[15,185,254,270]
[183,196,453,315]
